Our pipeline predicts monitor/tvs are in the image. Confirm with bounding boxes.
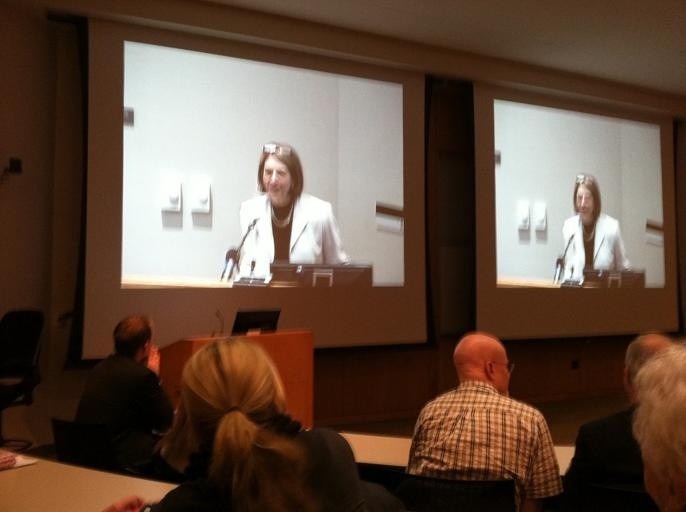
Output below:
[231,310,281,335]
[269,263,373,288]
[582,269,645,288]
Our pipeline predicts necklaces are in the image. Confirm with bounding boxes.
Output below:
[583,224,595,241]
[270,204,294,228]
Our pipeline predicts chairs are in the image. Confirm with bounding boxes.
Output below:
[1,309,46,453]
[50,415,666,512]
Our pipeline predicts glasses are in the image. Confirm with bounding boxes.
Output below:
[494,361,514,373]
[262,143,292,158]
[575,173,595,186]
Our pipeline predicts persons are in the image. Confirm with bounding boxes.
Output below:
[563,331,673,512]
[403,331,562,511]
[239,141,351,284]
[152,336,370,512]
[628,336,686,512]
[563,173,633,285]
[74,315,182,484]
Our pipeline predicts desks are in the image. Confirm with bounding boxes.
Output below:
[0,449,182,512]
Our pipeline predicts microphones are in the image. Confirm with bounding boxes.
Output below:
[554,257,565,285]
[562,234,575,277]
[215,307,225,336]
[221,249,240,281]
[250,261,255,277]
[571,267,574,277]
[237,218,259,272]
